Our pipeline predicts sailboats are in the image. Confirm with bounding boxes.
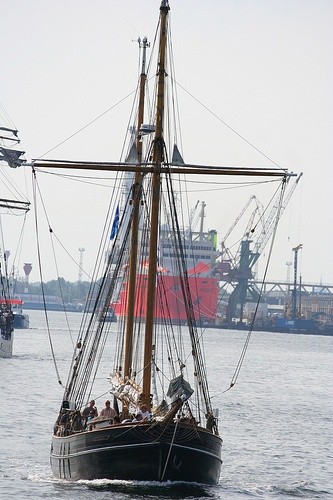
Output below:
[0,100,31,358]
[19,0,297,486]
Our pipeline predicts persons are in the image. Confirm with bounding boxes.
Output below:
[60,400,154,433]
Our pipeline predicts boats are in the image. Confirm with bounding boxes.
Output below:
[95,306,118,322]
[14,311,30,329]
[103,164,221,324]
